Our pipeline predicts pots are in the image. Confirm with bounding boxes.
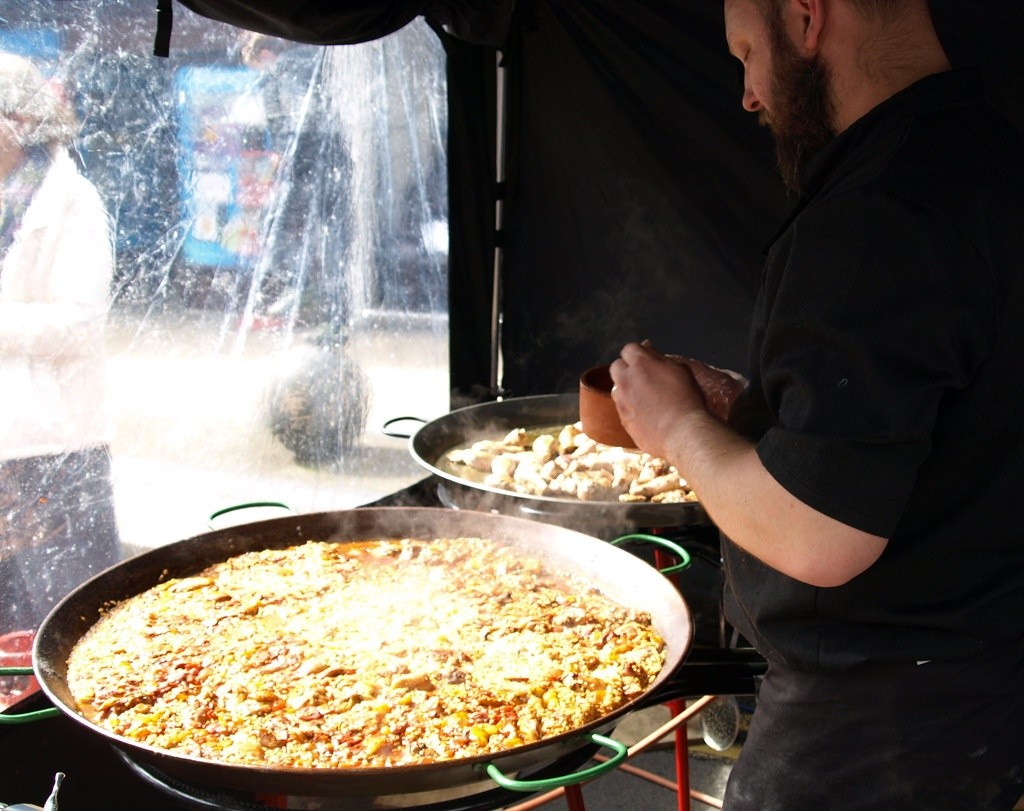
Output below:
[380,390,716,555]
[0,499,691,811]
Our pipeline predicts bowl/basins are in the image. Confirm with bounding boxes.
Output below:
[577,363,639,445]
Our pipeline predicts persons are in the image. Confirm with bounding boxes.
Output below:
[243,33,353,346]
[0,55,118,632]
[607,0,1024,811]
